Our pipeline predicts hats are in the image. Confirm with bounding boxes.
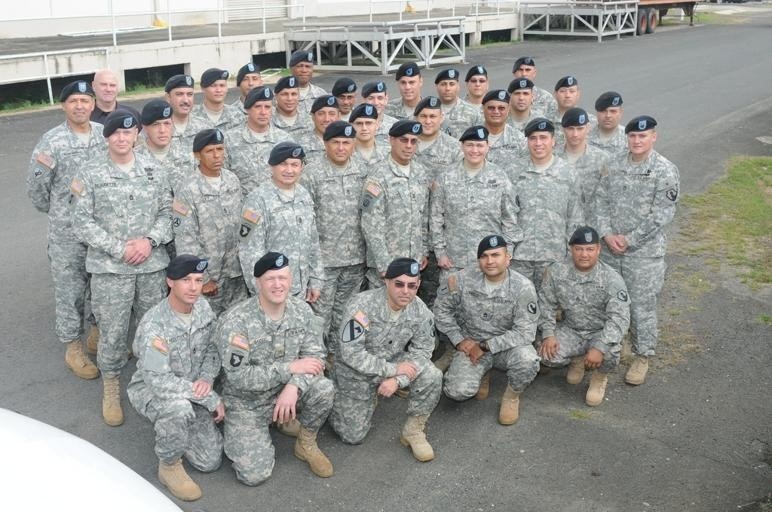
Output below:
[525,118,554,137]
[141,100,174,126]
[560,108,589,127]
[267,142,304,165]
[236,63,301,108]
[568,226,599,245]
[625,116,657,135]
[60,79,95,104]
[594,92,622,111]
[511,57,534,74]
[388,122,423,137]
[167,254,208,280]
[311,77,388,141]
[165,74,194,94]
[254,252,289,277]
[477,235,507,259]
[385,257,421,280]
[199,68,228,87]
[288,50,314,67]
[458,126,488,141]
[394,63,535,114]
[555,76,578,92]
[102,108,142,138]
[192,129,224,152]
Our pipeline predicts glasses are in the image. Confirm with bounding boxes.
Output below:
[394,282,418,290]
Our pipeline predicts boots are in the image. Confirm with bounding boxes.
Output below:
[566,357,585,385]
[585,368,608,406]
[66,338,99,379]
[498,384,520,425]
[625,357,649,385]
[158,462,201,501]
[294,426,334,478]
[400,416,434,462]
[102,378,123,426]
[86,325,133,360]
[276,414,300,436]
[474,371,489,401]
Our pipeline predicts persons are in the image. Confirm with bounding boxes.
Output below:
[323,258,443,461]
[66,51,631,426]
[28,70,143,379]
[126,254,225,501]
[214,252,335,486]
[586,115,679,384]
